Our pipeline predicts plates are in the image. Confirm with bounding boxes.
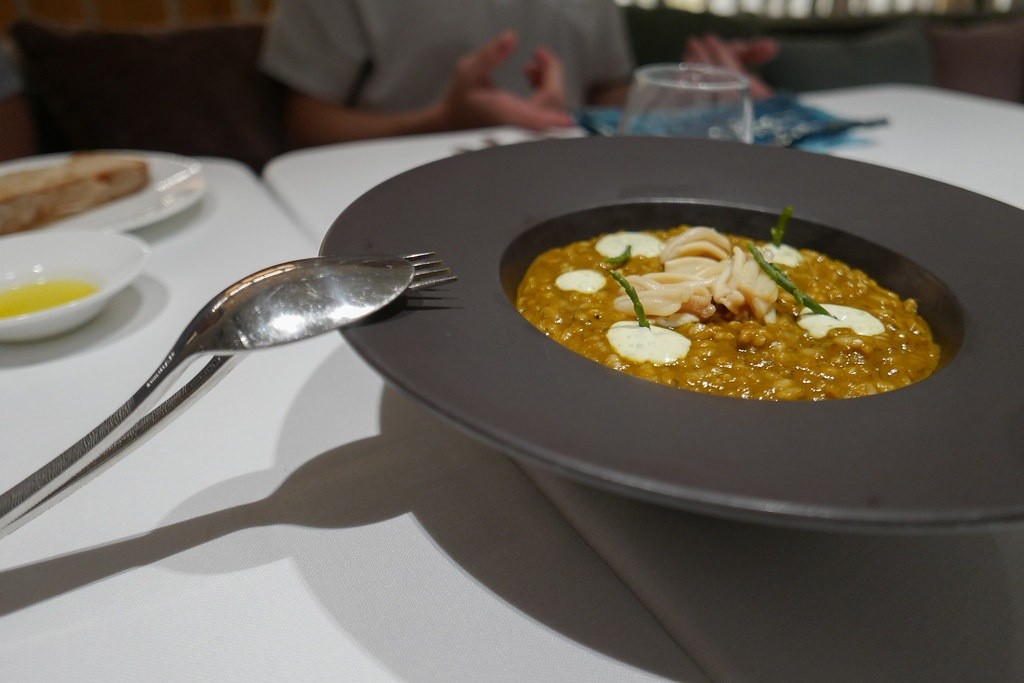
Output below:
[0,231,148,339]
[0,150,206,233]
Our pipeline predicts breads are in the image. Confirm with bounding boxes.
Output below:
[0,157,148,236]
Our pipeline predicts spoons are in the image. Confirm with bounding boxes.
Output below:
[0,257,415,529]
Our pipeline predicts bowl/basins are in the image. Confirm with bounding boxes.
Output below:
[319,137,1024,535]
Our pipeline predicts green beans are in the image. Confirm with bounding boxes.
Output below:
[608,269,650,329]
[748,244,832,317]
[605,247,631,264]
[772,205,792,246]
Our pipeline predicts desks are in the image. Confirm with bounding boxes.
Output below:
[0,86,1024,683]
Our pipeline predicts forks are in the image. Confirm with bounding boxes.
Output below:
[0,252,457,539]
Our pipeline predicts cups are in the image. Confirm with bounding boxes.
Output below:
[617,63,754,143]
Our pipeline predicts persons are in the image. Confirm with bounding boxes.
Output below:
[257,0,778,151]
